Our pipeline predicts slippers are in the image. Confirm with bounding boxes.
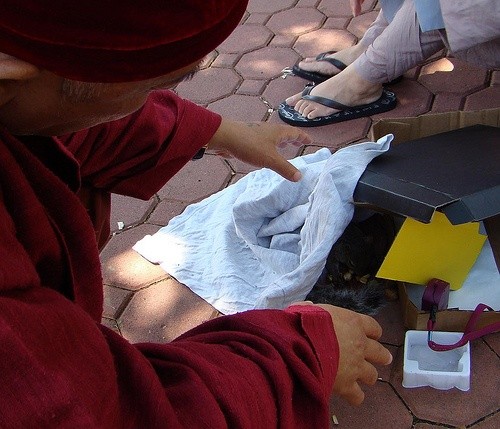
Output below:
[278,86,397,127]
[293,51,404,86]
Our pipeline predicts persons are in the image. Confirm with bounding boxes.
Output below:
[0,0,392,429]
[279,0,500,127]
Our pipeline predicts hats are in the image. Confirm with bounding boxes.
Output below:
[0,0,249,84]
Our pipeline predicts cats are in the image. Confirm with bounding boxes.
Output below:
[310,204,404,318]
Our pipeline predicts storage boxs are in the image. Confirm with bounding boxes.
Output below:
[352,107,500,343]
